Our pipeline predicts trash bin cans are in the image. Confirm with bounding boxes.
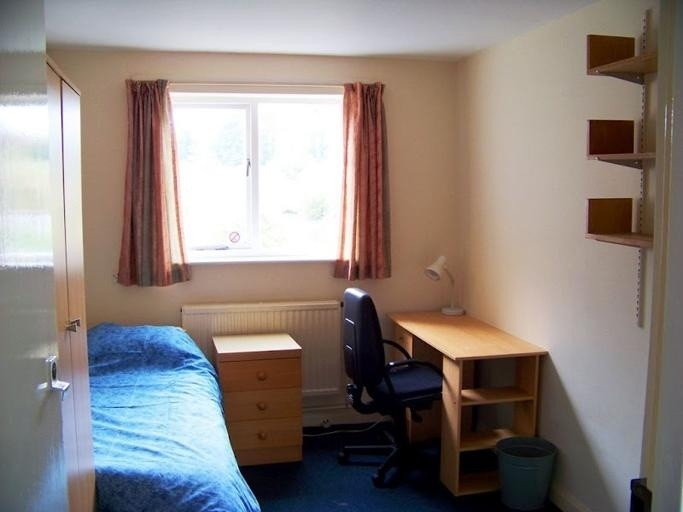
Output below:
[495,436,559,510]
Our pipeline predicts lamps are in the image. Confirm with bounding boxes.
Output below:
[424,254,464,316]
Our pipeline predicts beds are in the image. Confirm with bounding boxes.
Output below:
[84,323,263,512]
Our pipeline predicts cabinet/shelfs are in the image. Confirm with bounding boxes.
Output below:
[581,34,658,250]
[384,311,548,496]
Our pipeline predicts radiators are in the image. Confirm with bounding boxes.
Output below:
[180,299,343,396]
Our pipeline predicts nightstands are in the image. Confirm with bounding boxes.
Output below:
[210,333,304,467]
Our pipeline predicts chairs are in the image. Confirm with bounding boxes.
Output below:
[338,288,444,488]
